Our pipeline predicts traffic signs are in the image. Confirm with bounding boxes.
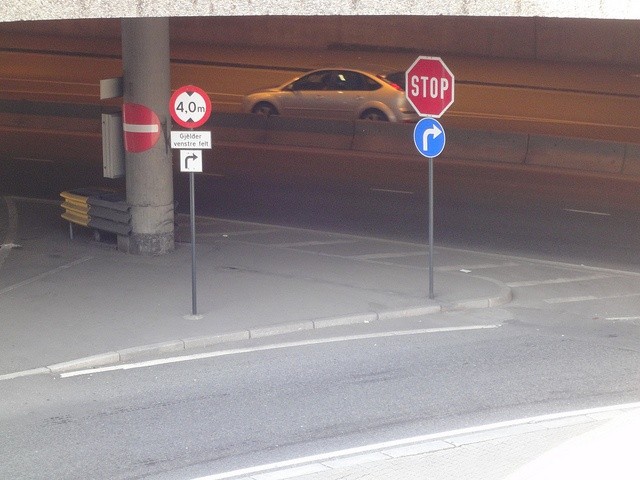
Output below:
[170,131,212,149]
[169,85,211,128]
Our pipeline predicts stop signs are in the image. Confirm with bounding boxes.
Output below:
[405,56,455,118]
[122,102,161,152]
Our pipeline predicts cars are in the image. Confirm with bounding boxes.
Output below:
[241,68,405,122]
